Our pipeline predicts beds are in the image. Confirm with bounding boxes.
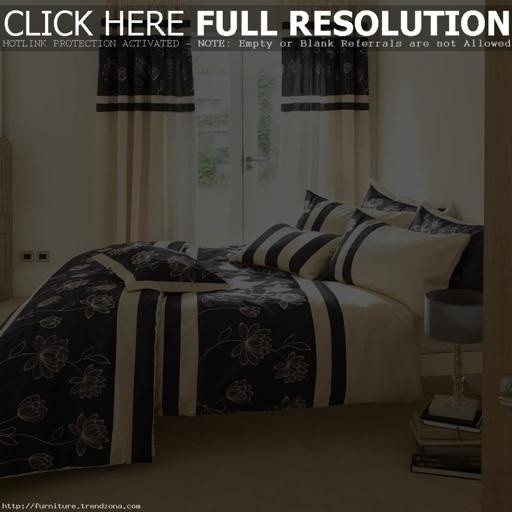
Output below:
[1,240,485,480]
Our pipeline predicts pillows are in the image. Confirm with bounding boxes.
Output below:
[89,179,485,320]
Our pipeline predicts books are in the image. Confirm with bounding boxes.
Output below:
[408,394,482,480]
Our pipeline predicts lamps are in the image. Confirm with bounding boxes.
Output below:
[420,290,483,432]
